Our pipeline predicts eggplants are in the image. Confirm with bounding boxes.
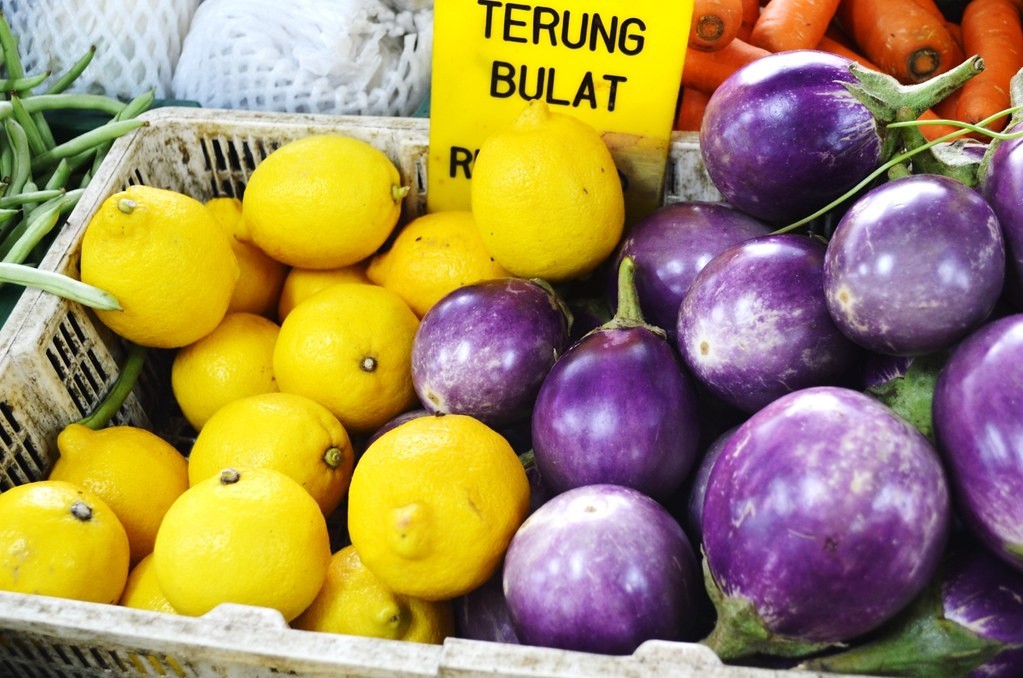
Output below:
[394,56,1023,677]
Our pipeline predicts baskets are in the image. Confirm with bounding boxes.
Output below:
[2,106,835,677]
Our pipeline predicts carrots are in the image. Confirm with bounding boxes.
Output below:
[676,0,1023,147]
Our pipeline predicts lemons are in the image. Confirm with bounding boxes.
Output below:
[0,98,624,644]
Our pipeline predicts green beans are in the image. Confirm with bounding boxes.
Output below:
[0,10,151,428]
